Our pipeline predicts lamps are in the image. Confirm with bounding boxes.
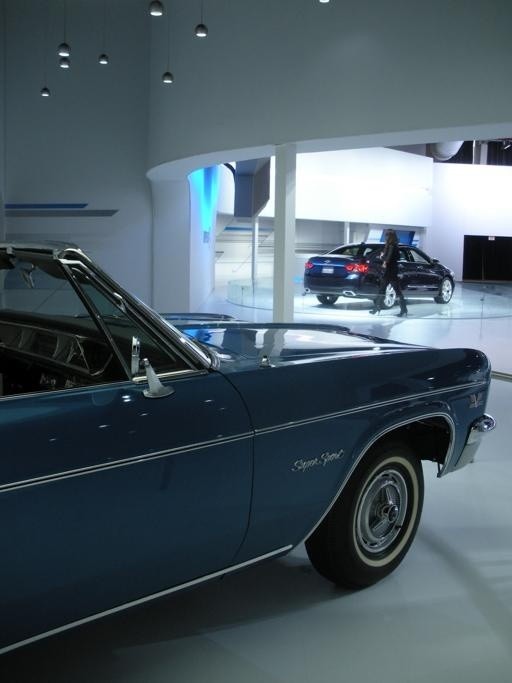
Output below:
[40,0,209,95]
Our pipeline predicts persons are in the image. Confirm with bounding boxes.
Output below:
[369,228,408,317]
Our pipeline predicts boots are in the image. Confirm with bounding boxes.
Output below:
[369,296,384,315]
[397,300,408,317]
